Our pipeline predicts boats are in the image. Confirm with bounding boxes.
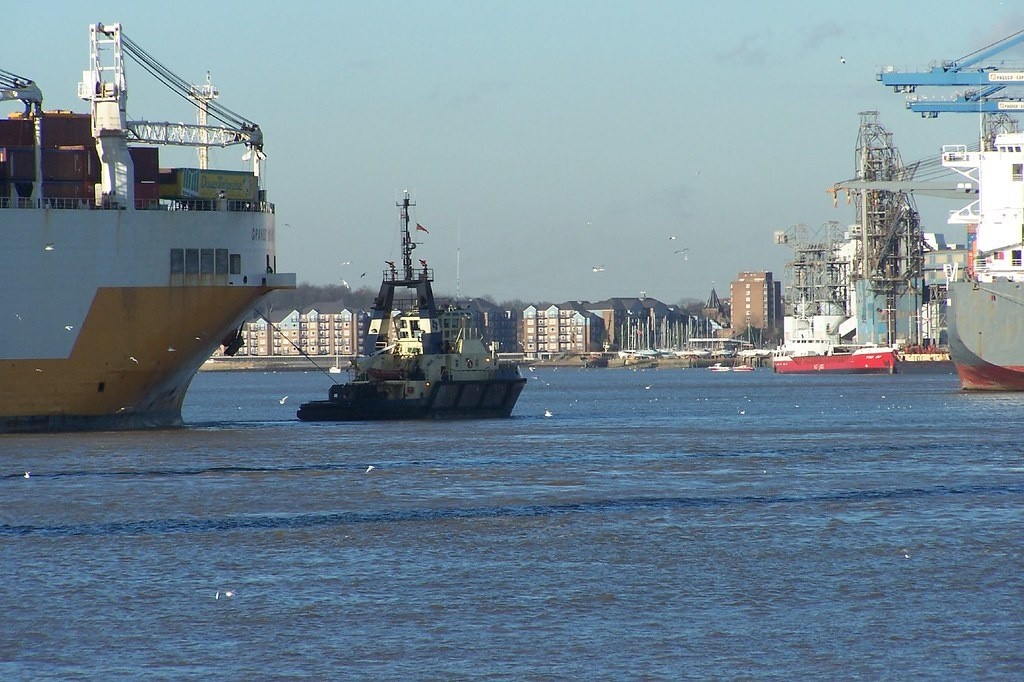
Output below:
[294,189,527,420]
[945,276,1024,392]
[707,362,730,373]
[772,342,896,376]
[733,365,755,372]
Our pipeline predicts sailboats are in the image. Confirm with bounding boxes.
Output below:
[618,311,781,361]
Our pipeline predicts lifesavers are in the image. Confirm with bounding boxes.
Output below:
[468,359,473,368]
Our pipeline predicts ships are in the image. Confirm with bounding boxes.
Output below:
[1,20,302,433]
